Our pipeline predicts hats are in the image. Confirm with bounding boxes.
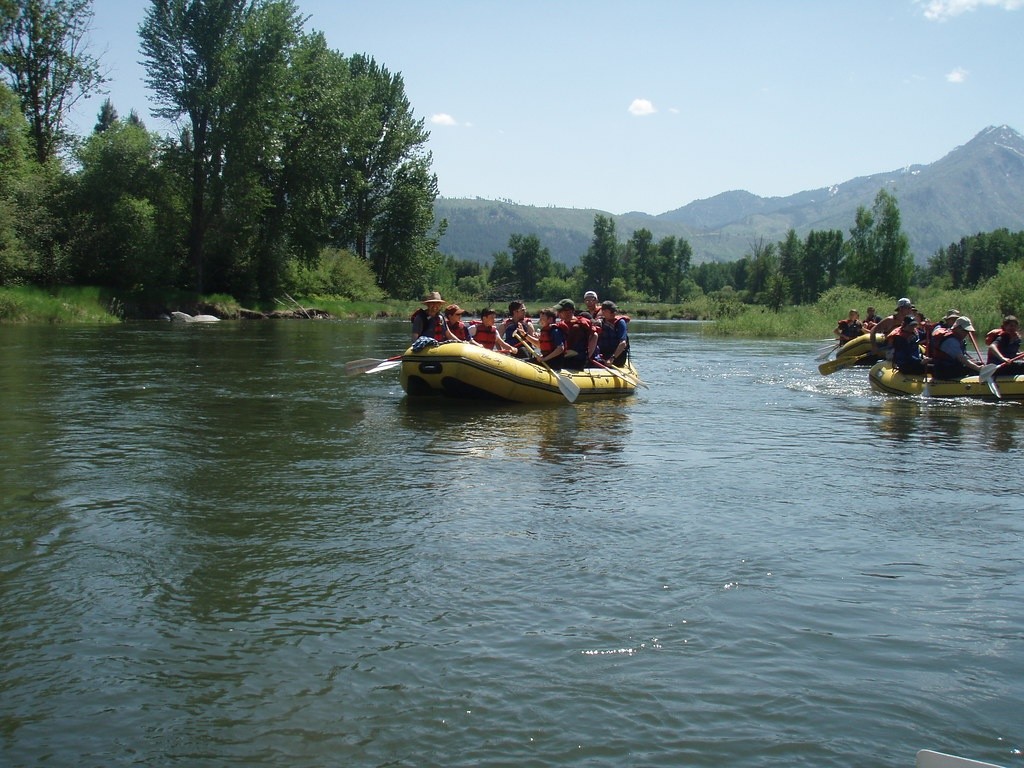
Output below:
[957,317,975,332]
[894,297,910,311]
[553,299,575,310]
[945,310,959,319]
[904,315,918,326]
[584,291,597,300]
[602,301,619,310]
[424,292,445,303]
[444,304,464,314]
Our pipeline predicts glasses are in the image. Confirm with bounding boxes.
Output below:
[584,300,596,303]
[607,309,617,314]
[516,308,526,312]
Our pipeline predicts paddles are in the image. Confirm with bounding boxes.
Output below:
[979,351,1024,383]
[609,363,650,390]
[818,345,893,377]
[814,337,840,362]
[345,353,403,377]
[968,331,1001,399]
[366,360,402,375]
[590,358,642,391]
[512,328,580,404]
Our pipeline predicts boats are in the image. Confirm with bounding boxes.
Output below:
[398,342,640,406]
[834,333,888,366]
[869,353,1024,399]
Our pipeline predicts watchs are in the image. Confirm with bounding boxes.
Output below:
[611,354,617,360]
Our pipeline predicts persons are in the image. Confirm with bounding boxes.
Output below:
[833,298,1024,380]
[411,290,630,369]
